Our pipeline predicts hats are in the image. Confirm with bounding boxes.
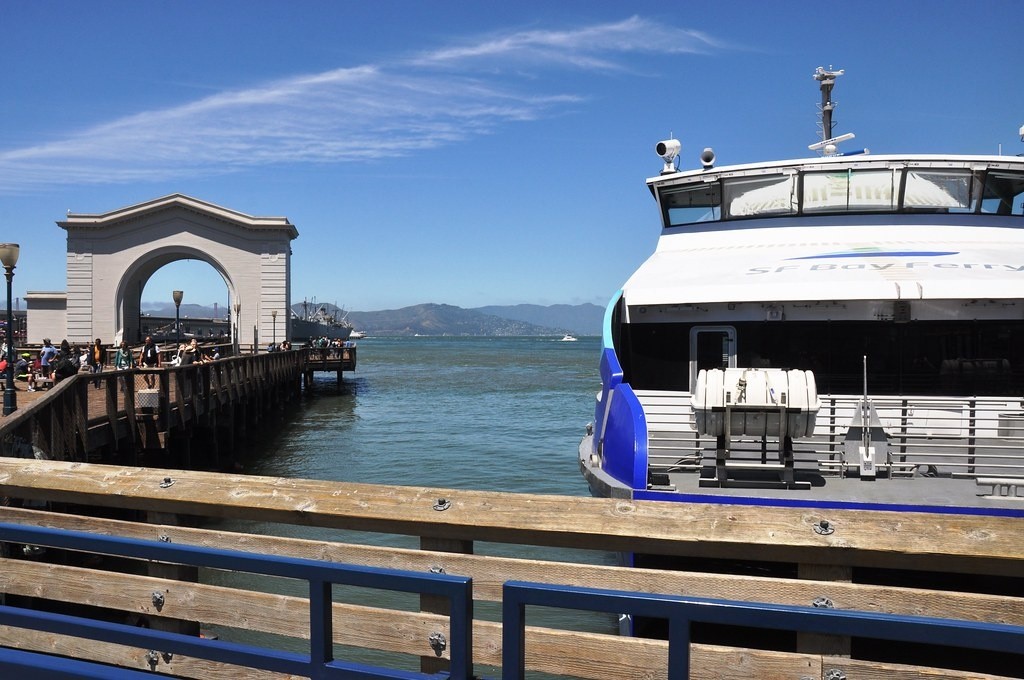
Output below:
[43,337,51,345]
[22,353,30,357]
[212,348,219,352]
[185,346,197,353]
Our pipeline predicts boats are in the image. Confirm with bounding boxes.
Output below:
[576,65,1024,680]
[291,297,353,342]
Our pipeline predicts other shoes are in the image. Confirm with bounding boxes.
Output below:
[94,384,100,390]
[28,386,40,392]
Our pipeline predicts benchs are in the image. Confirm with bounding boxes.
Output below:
[0,378,53,391]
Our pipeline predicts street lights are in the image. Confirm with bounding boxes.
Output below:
[234,303,241,357]
[0,242,20,416]
[173,290,184,367]
[272,311,277,352]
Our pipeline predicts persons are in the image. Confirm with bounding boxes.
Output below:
[267,340,291,353]
[87,338,107,390]
[139,336,161,389]
[0,336,80,392]
[114,341,133,391]
[304,335,352,359]
[171,339,220,397]
